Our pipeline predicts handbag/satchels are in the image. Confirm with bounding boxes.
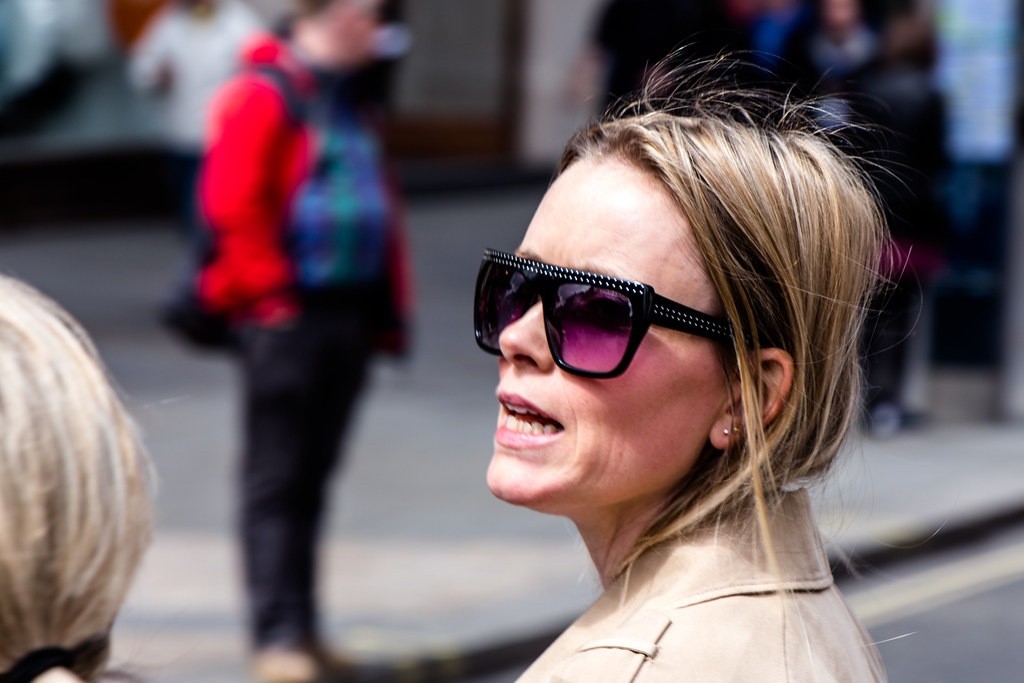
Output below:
[166,219,245,348]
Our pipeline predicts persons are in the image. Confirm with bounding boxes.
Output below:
[473,45,901,683]
[581,0,956,441]
[195,0,422,683]
[0,271,161,683]
[127,0,269,317]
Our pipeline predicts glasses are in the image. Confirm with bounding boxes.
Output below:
[473,247,755,378]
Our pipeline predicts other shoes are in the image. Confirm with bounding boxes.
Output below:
[250,639,319,683]
